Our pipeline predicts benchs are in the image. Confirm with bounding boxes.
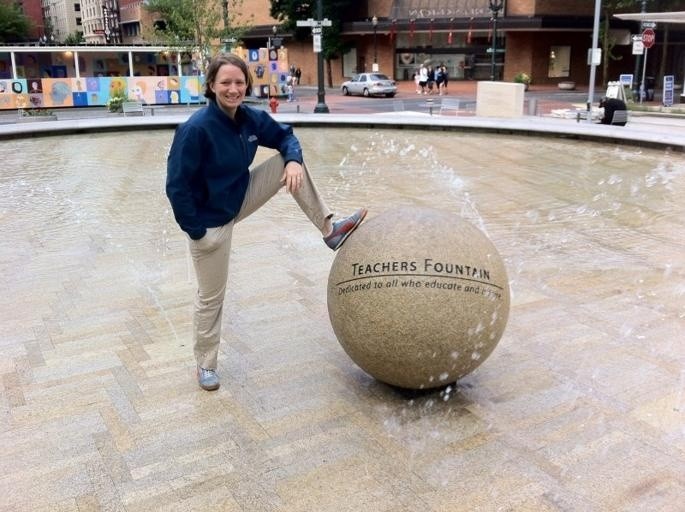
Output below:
[438,97,459,116]
[121,101,145,116]
[610,110,632,124]
[242,97,263,105]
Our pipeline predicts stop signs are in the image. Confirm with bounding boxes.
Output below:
[642,29,656,48]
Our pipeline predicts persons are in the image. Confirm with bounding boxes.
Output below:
[596,96,628,127]
[164,53,369,391]
[285,64,302,103]
[414,63,450,96]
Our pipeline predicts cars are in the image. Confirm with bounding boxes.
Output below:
[341,72,397,98]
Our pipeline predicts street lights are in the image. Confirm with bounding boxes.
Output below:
[272,26,278,39]
[488,0,503,81]
[371,16,378,72]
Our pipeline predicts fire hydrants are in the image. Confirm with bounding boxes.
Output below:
[270,98,279,113]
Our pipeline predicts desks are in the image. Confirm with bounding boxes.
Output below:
[144,106,165,115]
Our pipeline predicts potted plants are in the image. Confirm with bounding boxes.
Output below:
[514,71,532,91]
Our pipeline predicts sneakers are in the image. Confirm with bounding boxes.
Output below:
[196,366,222,390]
[322,205,371,251]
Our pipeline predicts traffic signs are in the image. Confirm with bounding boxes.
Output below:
[631,35,644,55]
